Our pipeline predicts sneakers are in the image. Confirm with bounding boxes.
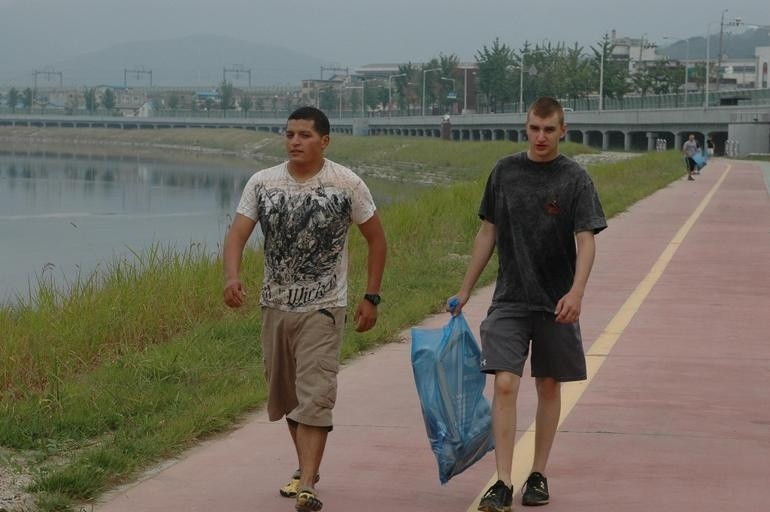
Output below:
[521,473,549,505]
[295,491,321,511]
[478,481,512,512]
[280,468,319,498]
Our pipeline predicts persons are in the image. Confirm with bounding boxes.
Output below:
[683,135,698,181]
[707,140,715,159]
[446,97,608,511]
[692,141,702,172]
[224,108,388,512]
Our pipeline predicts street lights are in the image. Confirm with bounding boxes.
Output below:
[389,73,407,117]
[423,68,443,116]
[599,35,631,110]
[704,17,744,108]
[520,50,545,112]
[339,82,355,121]
[362,79,376,119]
[664,36,688,107]
[441,77,455,95]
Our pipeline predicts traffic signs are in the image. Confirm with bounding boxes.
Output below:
[447,92,457,98]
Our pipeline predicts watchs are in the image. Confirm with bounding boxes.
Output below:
[363,292,384,306]
